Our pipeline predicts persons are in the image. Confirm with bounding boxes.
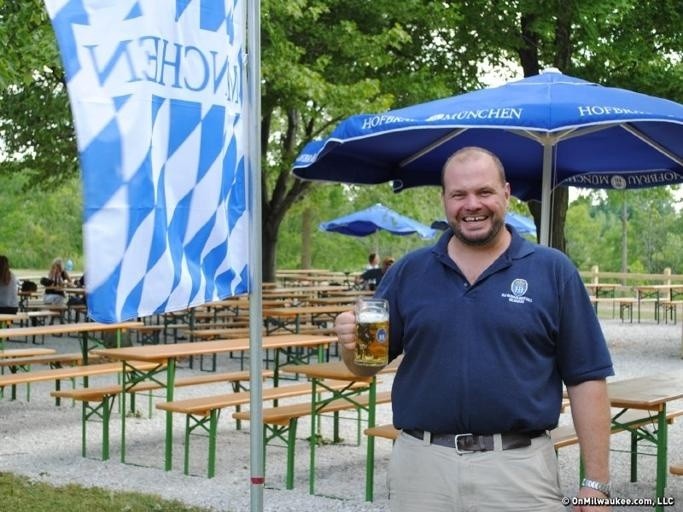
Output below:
[42,258,72,305]
[0,255,20,314]
[354,257,396,296]
[334,144,616,511]
[359,251,382,273]
[66,269,86,305]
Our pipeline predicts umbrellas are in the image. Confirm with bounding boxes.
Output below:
[291,73,683,246]
[323,203,440,270]
[430,210,536,245]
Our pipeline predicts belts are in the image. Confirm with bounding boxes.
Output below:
[404,429,532,453]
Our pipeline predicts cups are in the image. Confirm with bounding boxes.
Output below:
[350,299,390,367]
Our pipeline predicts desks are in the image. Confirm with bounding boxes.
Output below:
[276,358,403,507]
[635,284,683,323]
[0,312,60,347]
[266,305,359,333]
[95,336,338,469]
[582,282,622,316]
[0,322,143,411]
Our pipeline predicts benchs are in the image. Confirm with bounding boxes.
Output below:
[233,391,397,489]
[663,301,683,324]
[156,381,389,476]
[363,398,573,442]
[2,346,54,385]
[588,297,633,321]
[221,327,336,337]
[0,361,170,419]
[188,325,348,336]
[15,267,382,313]
[4,353,101,402]
[136,322,246,341]
[551,410,683,493]
[614,295,669,324]
[50,369,285,463]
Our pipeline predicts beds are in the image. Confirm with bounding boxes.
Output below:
[565,376,683,512]
